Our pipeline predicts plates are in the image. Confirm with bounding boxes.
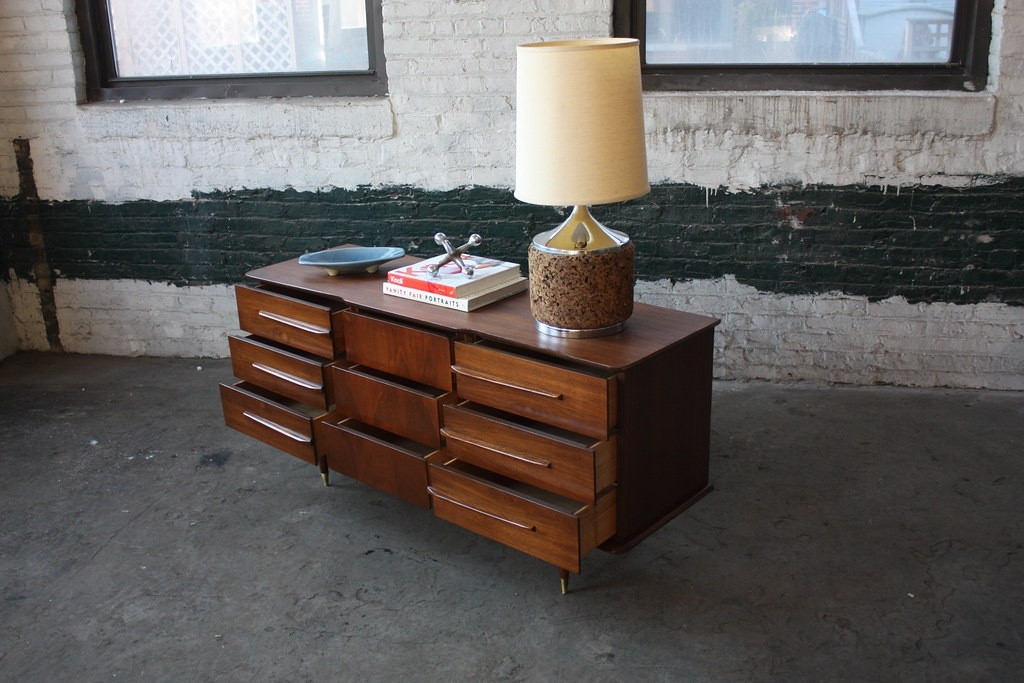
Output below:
[299,246,404,275]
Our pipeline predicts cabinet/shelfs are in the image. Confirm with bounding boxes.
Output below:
[216,243,723,597]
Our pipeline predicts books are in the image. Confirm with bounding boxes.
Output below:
[383,253,528,313]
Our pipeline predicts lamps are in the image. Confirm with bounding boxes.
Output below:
[512,38,652,339]
